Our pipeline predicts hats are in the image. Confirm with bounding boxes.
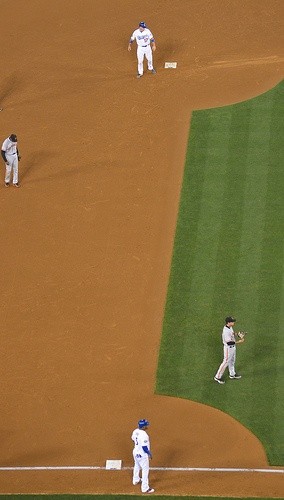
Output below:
[225,316,236,322]
[139,22,147,28]
[138,419,149,429]
[9,134,17,142]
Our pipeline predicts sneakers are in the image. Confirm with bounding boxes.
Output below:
[13,183,20,187]
[136,74,143,79]
[152,69,157,73]
[214,376,226,384]
[5,183,10,188]
[146,488,157,493]
[229,374,242,379]
[138,477,142,483]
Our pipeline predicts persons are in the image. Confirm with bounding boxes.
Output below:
[1,134,21,188]
[127,21,157,79]
[130,419,156,494]
[213,316,245,385]
